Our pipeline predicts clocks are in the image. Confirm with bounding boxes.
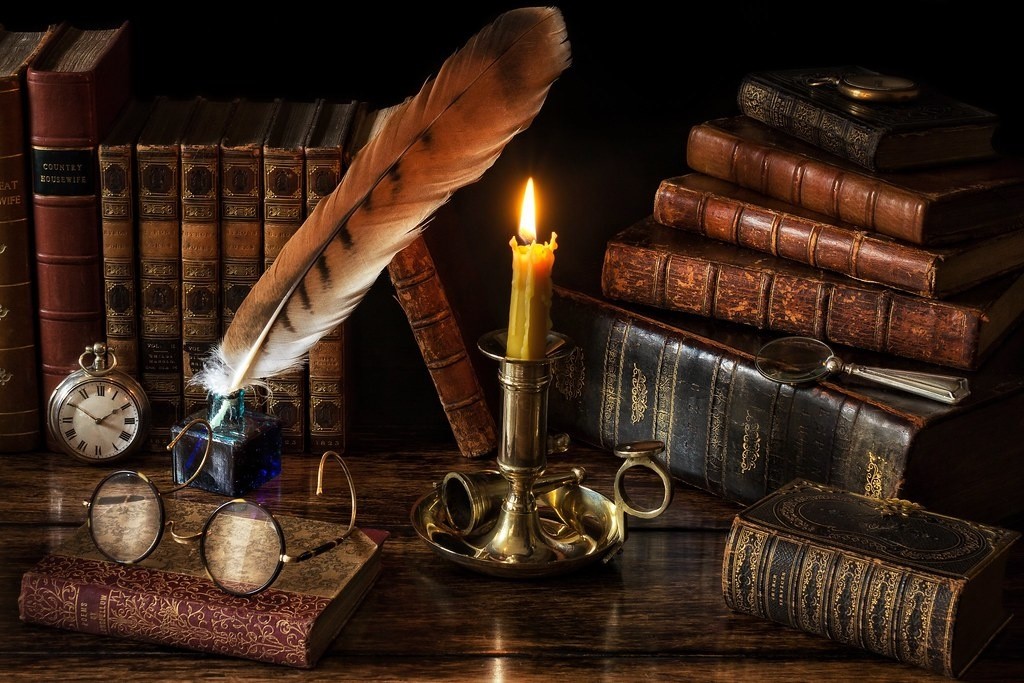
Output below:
[44,343,152,466]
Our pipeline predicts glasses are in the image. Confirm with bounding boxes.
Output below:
[81,418,358,598]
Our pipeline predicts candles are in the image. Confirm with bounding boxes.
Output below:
[504,173,558,358]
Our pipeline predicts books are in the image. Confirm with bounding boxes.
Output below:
[536,57,1024,679]
[345,98,500,461]
[20,494,394,672]
[0,19,387,466]
